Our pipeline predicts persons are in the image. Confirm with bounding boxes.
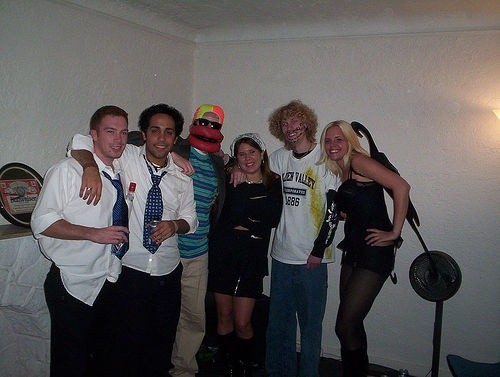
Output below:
[30,103,200,377]
[316,120,410,377]
[170,100,341,377]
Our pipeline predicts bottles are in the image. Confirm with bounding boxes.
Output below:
[125,182,136,202]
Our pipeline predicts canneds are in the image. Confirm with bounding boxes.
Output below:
[112,232,128,253]
[148,220,160,246]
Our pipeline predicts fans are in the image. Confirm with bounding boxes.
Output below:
[409,250,462,377]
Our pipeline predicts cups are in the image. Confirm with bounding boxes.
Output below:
[111,239,125,254]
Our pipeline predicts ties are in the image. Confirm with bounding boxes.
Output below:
[143,154,163,255]
[102,170,129,260]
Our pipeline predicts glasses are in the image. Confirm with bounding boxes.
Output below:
[194,118,222,131]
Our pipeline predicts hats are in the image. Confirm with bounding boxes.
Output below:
[194,104,224,125]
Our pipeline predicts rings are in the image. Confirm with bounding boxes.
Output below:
[87,188,92,190]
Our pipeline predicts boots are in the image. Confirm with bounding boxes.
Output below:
[217,329,236,377]
[236,334,259,377]
[341,346,370,377]
